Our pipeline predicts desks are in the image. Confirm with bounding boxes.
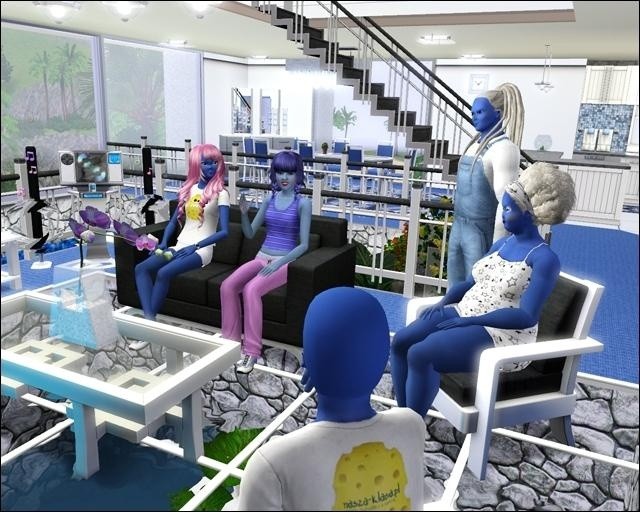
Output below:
[69,190,117,231]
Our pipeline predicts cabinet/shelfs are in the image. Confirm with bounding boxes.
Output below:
[582,65,630,104]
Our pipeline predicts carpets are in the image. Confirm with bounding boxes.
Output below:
[481,220,639,393]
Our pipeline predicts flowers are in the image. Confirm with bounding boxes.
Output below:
[67,206,173,297]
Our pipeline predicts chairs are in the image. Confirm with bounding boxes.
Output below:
[242,136,424,210]
[405,267,605,481]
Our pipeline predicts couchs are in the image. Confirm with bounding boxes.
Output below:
[115,198,359,348]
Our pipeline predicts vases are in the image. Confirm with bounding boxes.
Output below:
[50,257,117,352]
